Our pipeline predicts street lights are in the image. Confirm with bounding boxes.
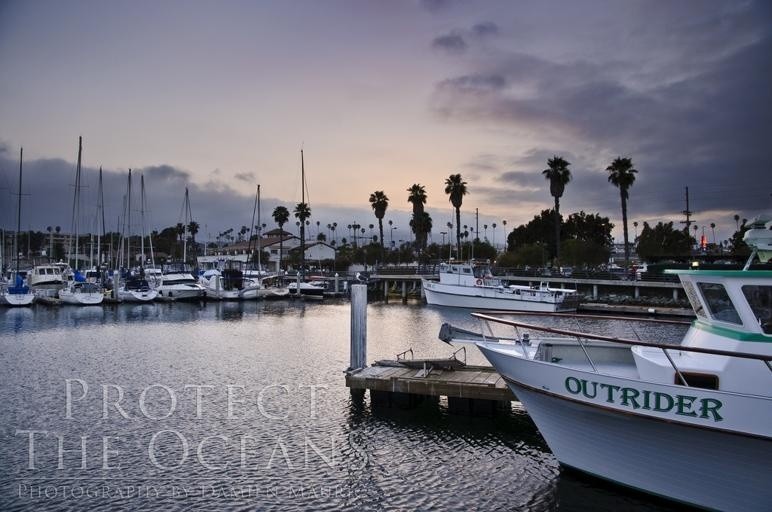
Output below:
[711,222,716,245]
[213,217,508,264]
[633,221,638,242]
[692,224,698,248]
[734,214,740,231]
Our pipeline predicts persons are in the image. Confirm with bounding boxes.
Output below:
[629,260,638,280]
[524,255,560,277]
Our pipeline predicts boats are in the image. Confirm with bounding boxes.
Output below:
[469,267,772,512]
[420,258,581,316]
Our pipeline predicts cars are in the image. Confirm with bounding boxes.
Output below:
[520,264,644,280]
